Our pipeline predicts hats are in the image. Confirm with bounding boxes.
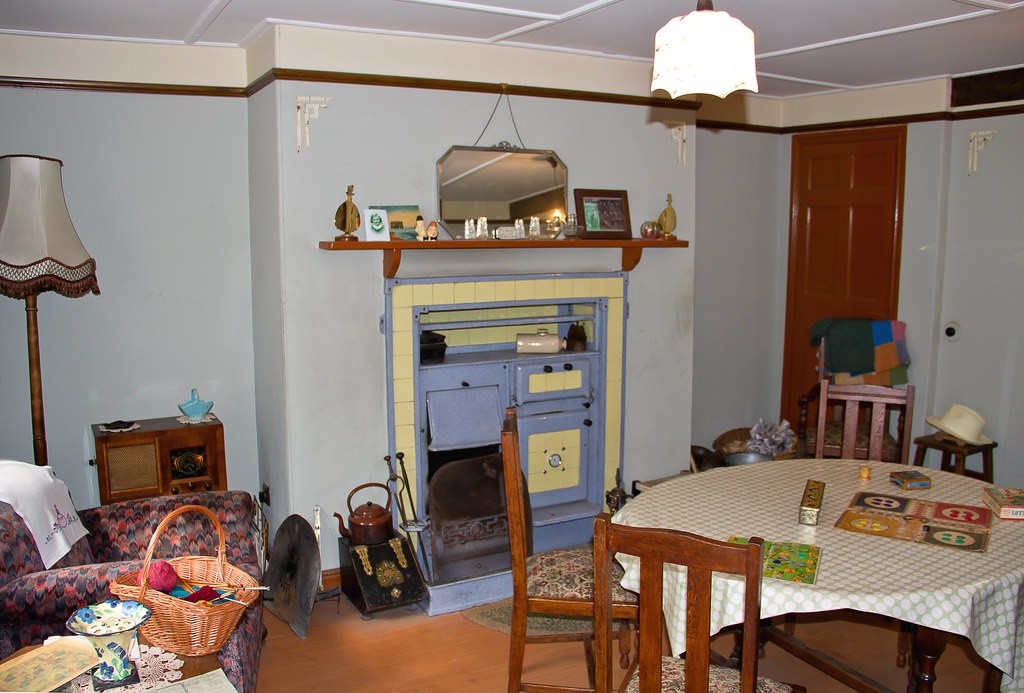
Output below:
[926,405,993,446]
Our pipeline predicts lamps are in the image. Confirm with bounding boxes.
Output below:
[650,0,760,98]
[0,153,101,467]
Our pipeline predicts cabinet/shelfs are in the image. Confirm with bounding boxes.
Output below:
[91,412,229,507]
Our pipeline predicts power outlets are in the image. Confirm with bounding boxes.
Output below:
[263,483,271,508]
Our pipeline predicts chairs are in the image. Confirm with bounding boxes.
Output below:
[815,379,915,465]
[592,510,807,693]
[502,407,642,693]
[795,317,912,463]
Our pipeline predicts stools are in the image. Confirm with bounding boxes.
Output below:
[914,433,999,485]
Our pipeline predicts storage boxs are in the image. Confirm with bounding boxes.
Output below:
[797,479,826,524]
[890,470,931,492]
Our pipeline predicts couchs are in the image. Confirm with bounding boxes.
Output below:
[0,460,269,693]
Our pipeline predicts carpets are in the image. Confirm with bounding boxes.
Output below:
[460,594,628,636]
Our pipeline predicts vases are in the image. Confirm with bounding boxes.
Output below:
[63,599,152,687]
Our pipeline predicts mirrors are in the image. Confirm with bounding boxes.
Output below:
[437,143,568,239]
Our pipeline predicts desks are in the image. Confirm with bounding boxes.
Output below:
[611,457,1024,693]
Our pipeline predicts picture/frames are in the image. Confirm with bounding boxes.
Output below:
[573,188,633,239]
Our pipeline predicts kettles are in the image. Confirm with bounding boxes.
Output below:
[333,482,393,545]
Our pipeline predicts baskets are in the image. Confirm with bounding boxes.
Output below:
[109,504,260,656]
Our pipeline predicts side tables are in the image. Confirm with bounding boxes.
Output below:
[0,644,227,693]
